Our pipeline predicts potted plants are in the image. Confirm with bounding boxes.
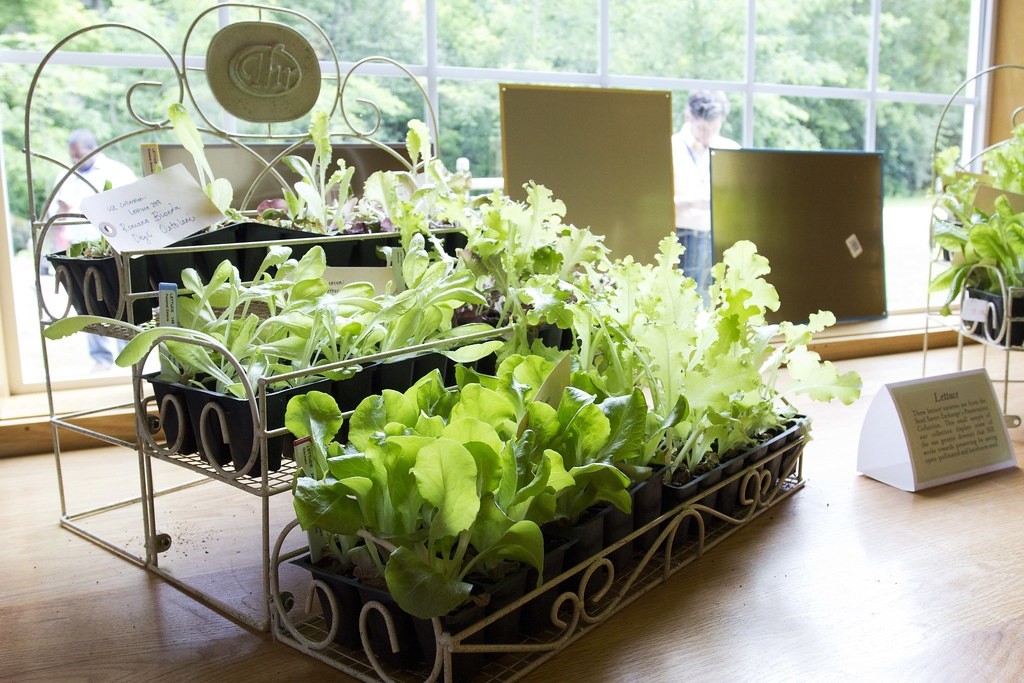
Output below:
[46,99,466,313]
[47,176,610,477]
[929,124,1024,347]
[291,244,807,683]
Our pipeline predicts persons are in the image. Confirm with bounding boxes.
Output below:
[671,88,742,310]
[51,130,137,376]
